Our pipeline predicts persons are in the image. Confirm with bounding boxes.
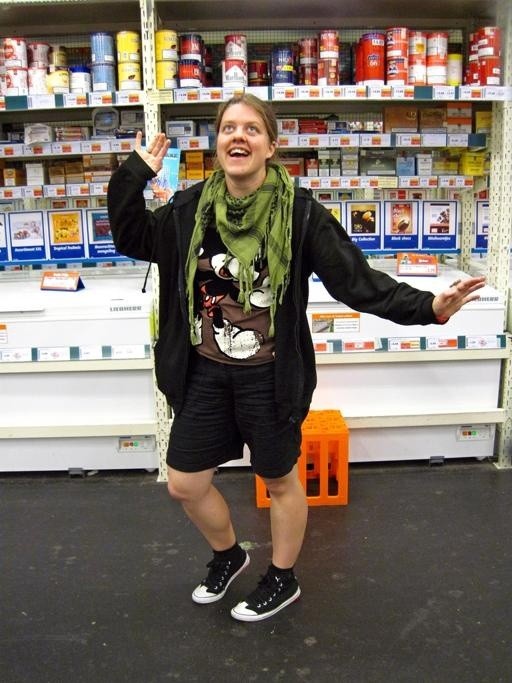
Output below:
[106,92,489,624]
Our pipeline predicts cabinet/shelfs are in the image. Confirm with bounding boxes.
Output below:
[150,0,511,296]
[1,2,147,268]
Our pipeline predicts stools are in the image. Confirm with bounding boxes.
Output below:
[255,409,350,509]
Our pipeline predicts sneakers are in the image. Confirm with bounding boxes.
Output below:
[192,549,251,605]
[230,572,301,622]
